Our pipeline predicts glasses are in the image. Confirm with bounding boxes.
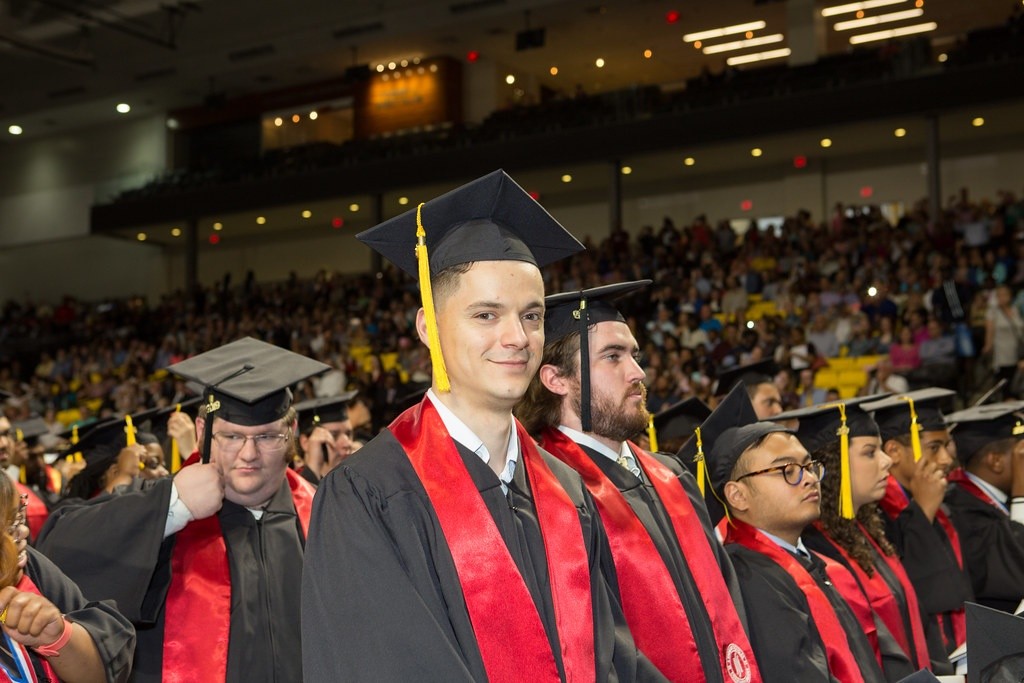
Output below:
[202,419,289,457]
[0,488,32,548]
[720,458,830,493]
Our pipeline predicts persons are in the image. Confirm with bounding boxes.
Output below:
[1,466,139,683]
[40,384,306,682]
[515,308,762,682]
[296,216,656,681]
[719,422,893,683]
[0,266,433,509]
[544,180,1022,464]
[944,406,1024,682]
[794,408,954,682]
[874,399,1000,683]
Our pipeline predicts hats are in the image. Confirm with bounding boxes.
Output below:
[711,357,785,396]
[670,381,793,498]
[854,385,956,439]
[136,393,204,441]
[59,400,163,472]
[651,393,710,445]
[939,398,1023,455]
[164,336,331,430]
[362,167,588,279]
[529,272,660,350]
[763,389,898,456]
[290,386,358,426]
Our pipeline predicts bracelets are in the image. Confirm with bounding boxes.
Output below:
[32,612,74,657]
